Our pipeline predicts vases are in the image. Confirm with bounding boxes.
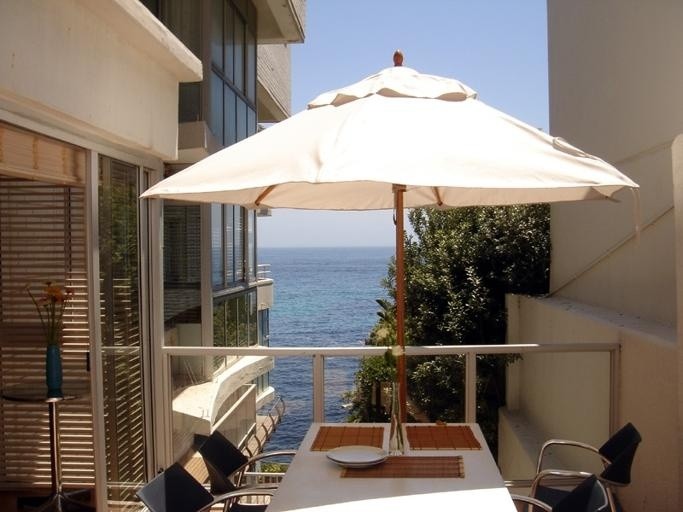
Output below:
[44,339,65,395]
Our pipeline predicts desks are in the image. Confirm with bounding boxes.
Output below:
[2,382,94,510]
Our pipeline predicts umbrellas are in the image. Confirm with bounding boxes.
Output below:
[137,50,640,422]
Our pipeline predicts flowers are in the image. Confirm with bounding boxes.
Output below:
[20,278,75,343]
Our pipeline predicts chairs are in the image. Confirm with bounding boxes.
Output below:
[136,461,275,512]
[528,424,641,511]
[512,472,596,512]
[198,429,297,509]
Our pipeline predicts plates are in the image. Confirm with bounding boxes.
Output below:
[325,445,391,469]
